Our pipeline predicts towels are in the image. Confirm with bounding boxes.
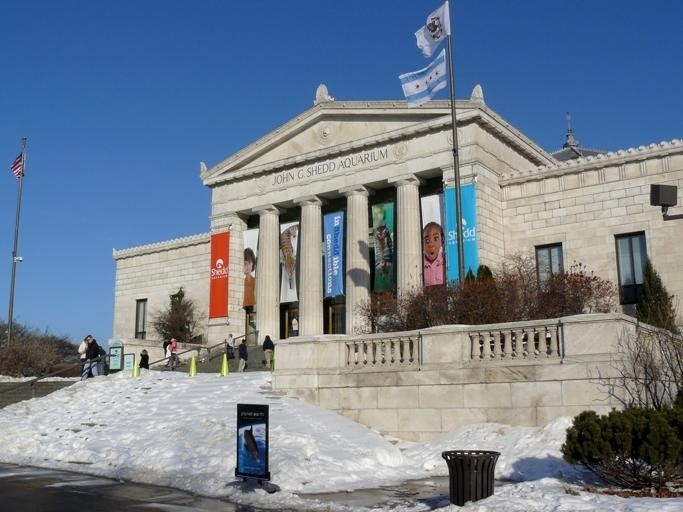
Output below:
[442,450,501,507]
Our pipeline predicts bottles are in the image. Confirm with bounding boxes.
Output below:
[170,338,176,341]
[242,339,246,343]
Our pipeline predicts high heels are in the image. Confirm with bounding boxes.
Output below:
[220,354,228,376]
[188,355,196,377]
[132,360,140,377]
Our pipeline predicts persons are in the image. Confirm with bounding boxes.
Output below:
[163,337,173,363]
[227,334,235,359]
[138,349,149,376]
[241,247,255,308]
[262,335,273,369]
[291,315,298,336]
[237,339,248,373]
[79,337,92,378]
[164,341,175,367]
[98,346,106,376]
[170,338,180,367]
[422,222,443,288]
[80,334,100,380]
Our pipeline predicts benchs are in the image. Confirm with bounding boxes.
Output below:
[413,0,451,58]
[397,46,451,107]
[10,153,24,180]
